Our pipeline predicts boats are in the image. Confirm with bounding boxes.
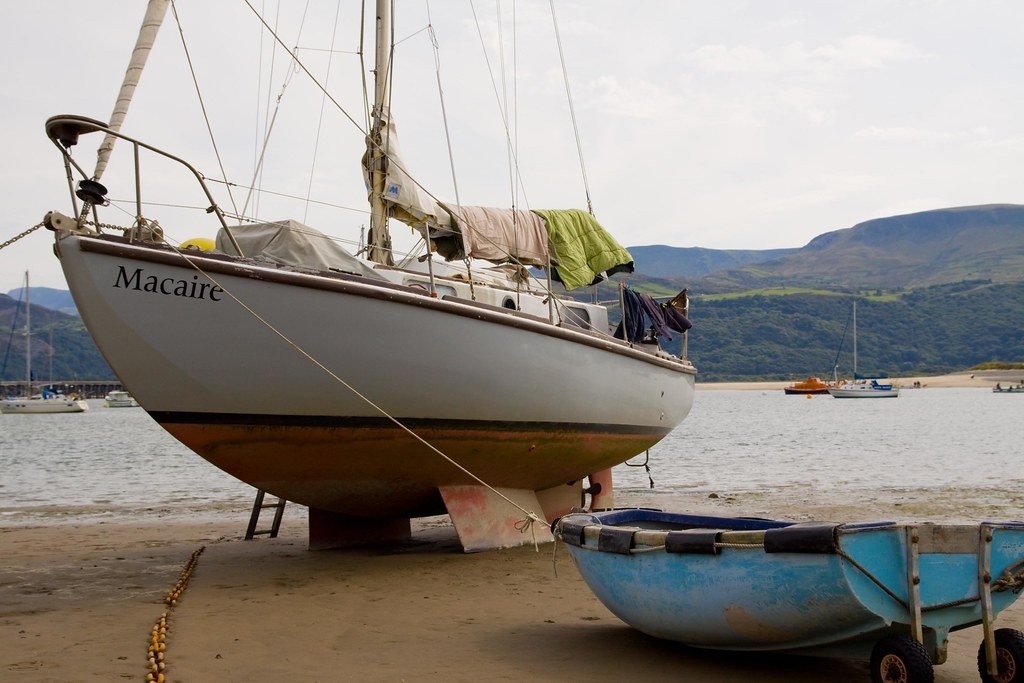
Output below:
[105,390,140,408]
[41,2,699,557]
[551,502,1024,664]
[784,365,849,394]
[991,386,1024,393]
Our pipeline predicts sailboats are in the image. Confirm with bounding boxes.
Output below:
[0,269,89,413]
[827,300,899,398]
